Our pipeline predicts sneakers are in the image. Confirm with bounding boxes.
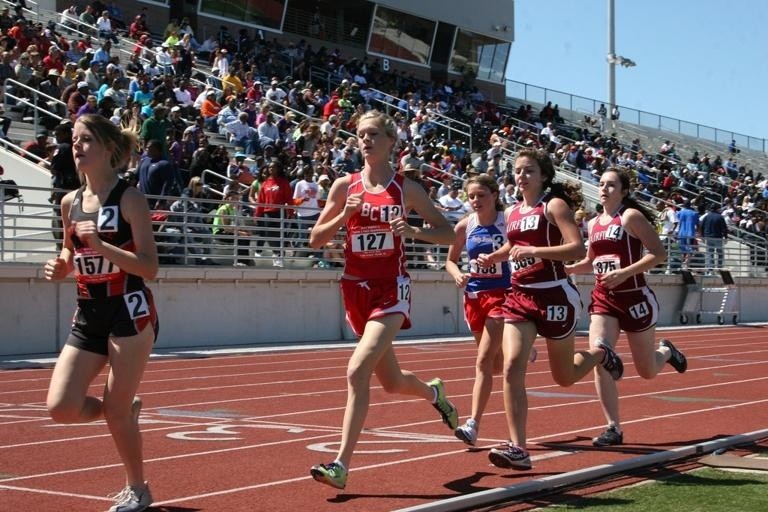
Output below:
[128,394,142,425]
[106,479,153,511]
[591,424,624,446]
[528,346,537,364]
[595,336,624,380]
[310,462,348,488]
[488,441,532,470]
[427,377,459,430]
[454,418,479,446]
[658,338,688,374]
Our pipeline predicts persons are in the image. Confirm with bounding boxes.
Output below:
[477,147,626,471]
[2,2,766,272]
[41,111,162,512]
[307,108,459,488]
[445,172,535,448]
[561,167,687,446]
